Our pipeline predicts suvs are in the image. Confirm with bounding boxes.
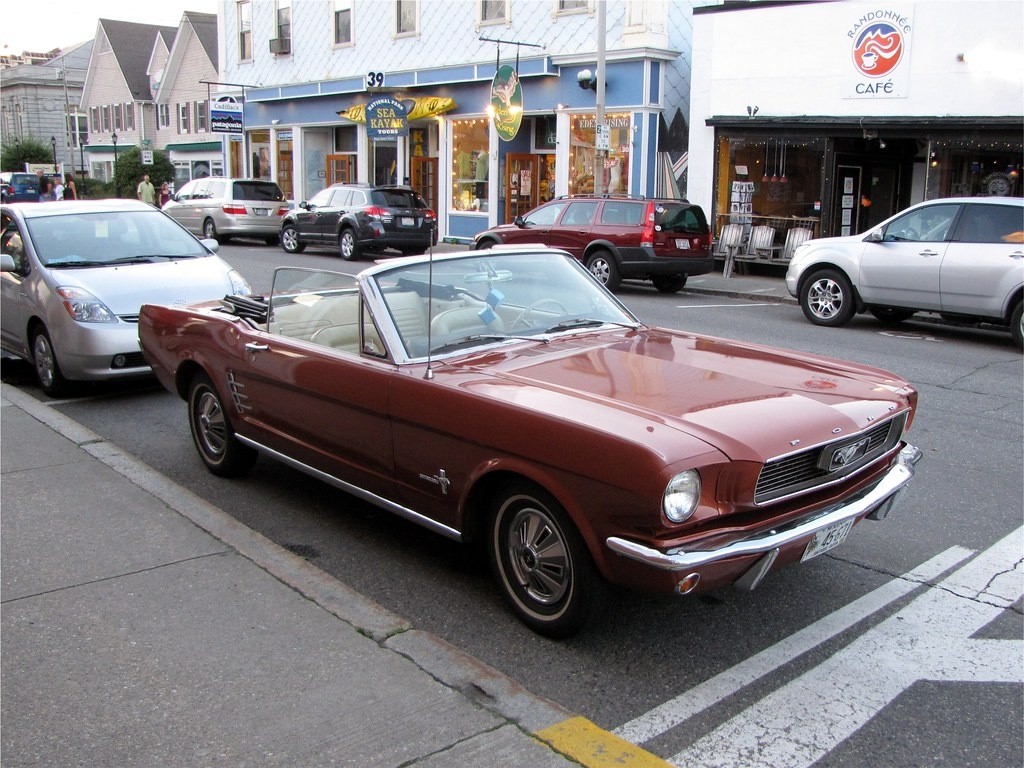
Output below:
[469,195,714,295]
[278,182,439,262]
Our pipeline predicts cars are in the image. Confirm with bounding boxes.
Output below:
[0,178,15,204]
[0,200,254,399]
[784,196,1024,353]
[139,245,924,643]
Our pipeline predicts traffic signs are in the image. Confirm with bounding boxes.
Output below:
[142,150,153,165]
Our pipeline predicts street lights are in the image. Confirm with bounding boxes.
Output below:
[14,137,21,172]
[112,133,122,199]
[78,136,87,195]
[50,136,57,177]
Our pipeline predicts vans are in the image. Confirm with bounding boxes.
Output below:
[162,176,291,246]
[0,172,40,202]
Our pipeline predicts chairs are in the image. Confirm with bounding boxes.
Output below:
[430,307,502,338]
[793,219,813,231]
[311,323,387,360]
[2,233,24,271]
[766,214,789,242]
[708,224,814,278]
[603,211,619,224]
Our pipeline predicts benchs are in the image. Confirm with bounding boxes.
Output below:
[267,291,462,346]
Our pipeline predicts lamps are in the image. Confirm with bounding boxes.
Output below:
[762,141,788,183]
[577,68,607,93]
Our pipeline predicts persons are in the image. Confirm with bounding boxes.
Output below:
[63,173,78,200]
[52,177,65,201]
[159,182,172,209]
[137,174,156,206]
[35,169,52,202]
[476,149,489,181]
